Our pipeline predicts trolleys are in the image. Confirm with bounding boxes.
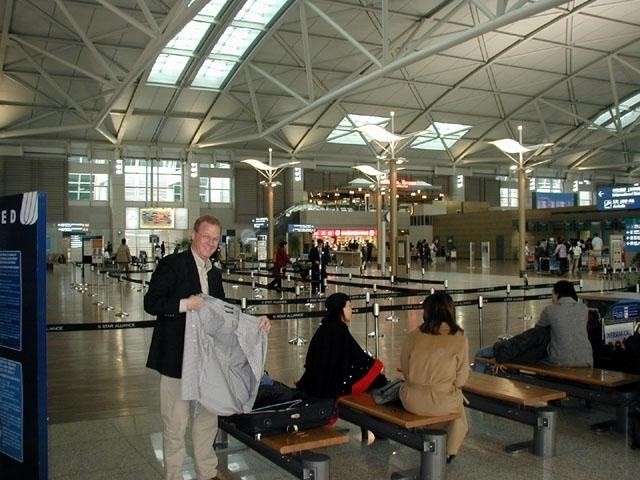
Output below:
[539,256,569,276]
[287,256,311,279]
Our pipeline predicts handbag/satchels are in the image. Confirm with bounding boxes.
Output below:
[371,379,404,404]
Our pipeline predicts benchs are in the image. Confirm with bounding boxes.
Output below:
[396,361,566,460]
[333,383,462,478]
[495,354,639,436]
[220,392,355,479]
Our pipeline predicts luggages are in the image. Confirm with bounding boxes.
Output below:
[216,369,336,442]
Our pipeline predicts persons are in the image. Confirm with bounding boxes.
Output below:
[275,239,375,293]
[140,215,274,480]
[396,290,472,466]
[104,238,131,282]
[302,292,391,444]
[525,233,604,276]
[409,239,454,267]
[536,280,594,411]
[154,240,165,264]
[471,325,551,375]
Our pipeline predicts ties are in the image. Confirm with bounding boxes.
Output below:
[319,247,320,254]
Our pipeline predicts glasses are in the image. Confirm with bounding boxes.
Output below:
[196,232,221,242]
